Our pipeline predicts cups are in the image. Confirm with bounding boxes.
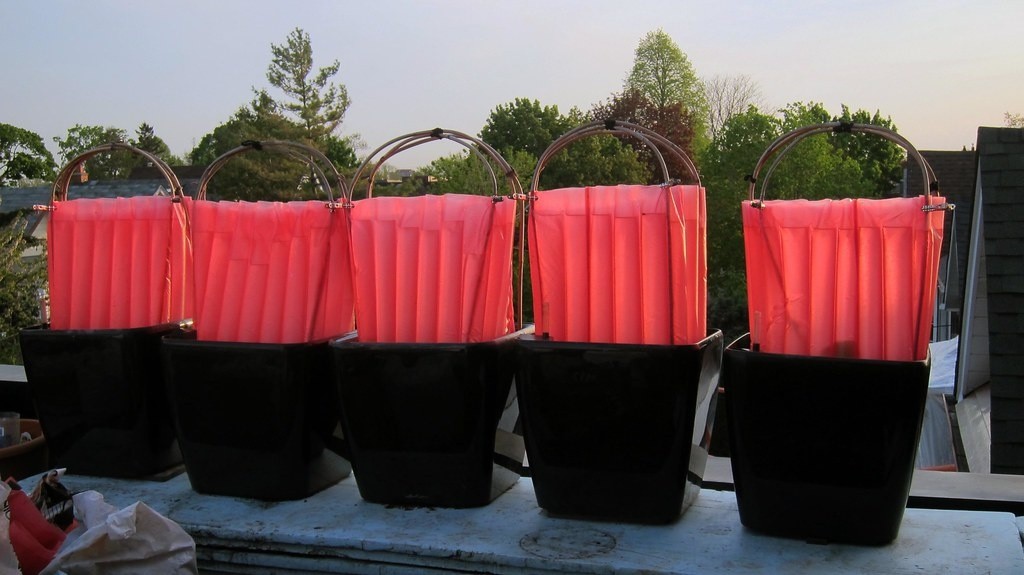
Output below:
[0,413,22,447]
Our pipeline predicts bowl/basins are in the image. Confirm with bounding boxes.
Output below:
[1,419,45,476]
